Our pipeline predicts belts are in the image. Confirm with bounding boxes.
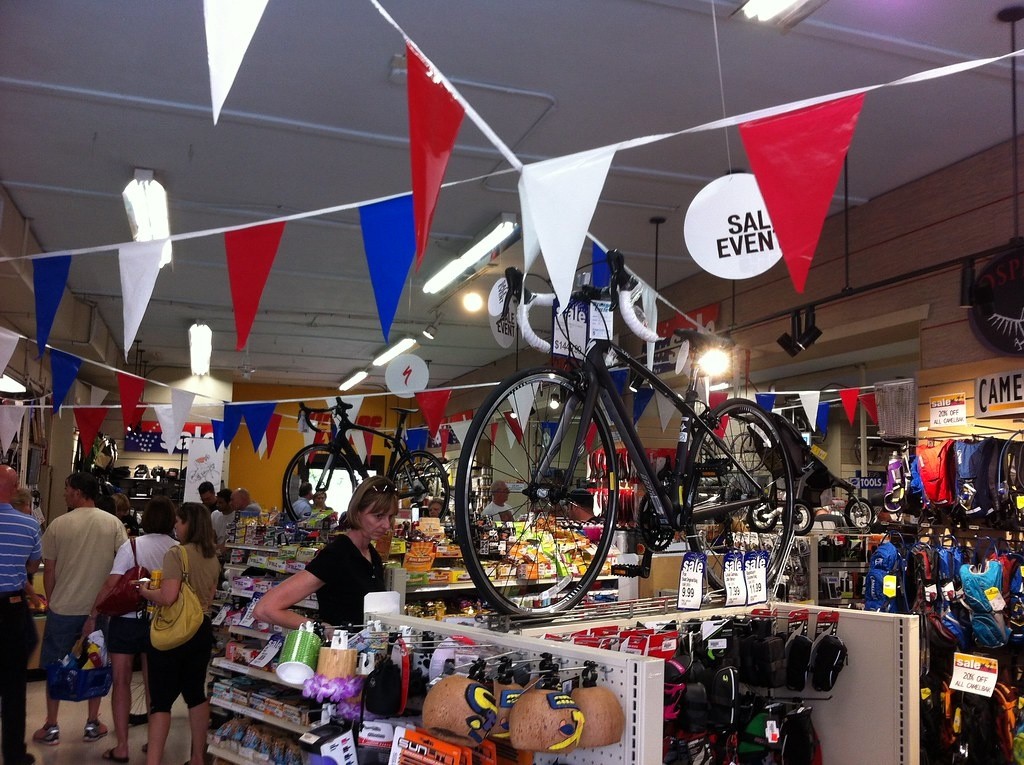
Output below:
[10,596,22,603]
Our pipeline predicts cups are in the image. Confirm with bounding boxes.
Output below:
[316,648,358,680]
[276,630,321,684]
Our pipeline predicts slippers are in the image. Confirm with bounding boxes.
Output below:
[103,747,129,764]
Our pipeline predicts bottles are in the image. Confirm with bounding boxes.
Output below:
[580,520,604,542]
[616,527,628,553]
[626,527,637,553]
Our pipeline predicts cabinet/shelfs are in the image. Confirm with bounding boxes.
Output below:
[111,476,185,537]
[207,426,1024,765]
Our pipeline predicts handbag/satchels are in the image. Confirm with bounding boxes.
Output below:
[149,545,205,650]
[96,537,150,617]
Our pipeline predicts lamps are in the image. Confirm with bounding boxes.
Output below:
[338,213,519,391]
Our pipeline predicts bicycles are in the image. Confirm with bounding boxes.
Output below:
[453,247,793,620]
[281,396,452,533]
[128,655,148,725]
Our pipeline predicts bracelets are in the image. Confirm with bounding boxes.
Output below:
[89,615,97,620]
[313,620,323,629]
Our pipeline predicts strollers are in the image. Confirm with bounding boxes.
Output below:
[730,410,875,532]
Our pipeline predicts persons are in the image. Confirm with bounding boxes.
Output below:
[481,480,515,521]
[111,493,139,538]
[292,482,313,519]
[563,489,594,520]
[199,482,262,590]
[138,503,221,765]
[429,498,445,518]
[32,472,128,746]
[252,476,400,647]
[311,491,334,516]
[81,497,181,763]
[0,465,42,765]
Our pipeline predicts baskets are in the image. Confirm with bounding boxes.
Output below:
[45,663,113,701]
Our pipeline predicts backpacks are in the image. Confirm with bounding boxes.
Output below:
[863,437,1024,765]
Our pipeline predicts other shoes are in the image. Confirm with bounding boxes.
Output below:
[3,752,37,765]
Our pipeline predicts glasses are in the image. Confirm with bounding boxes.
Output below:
[181,502,188,516]
[366,483,399,497]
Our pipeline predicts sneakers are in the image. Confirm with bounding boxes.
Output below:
[83,718,109,741]
[33,723,60,744]
